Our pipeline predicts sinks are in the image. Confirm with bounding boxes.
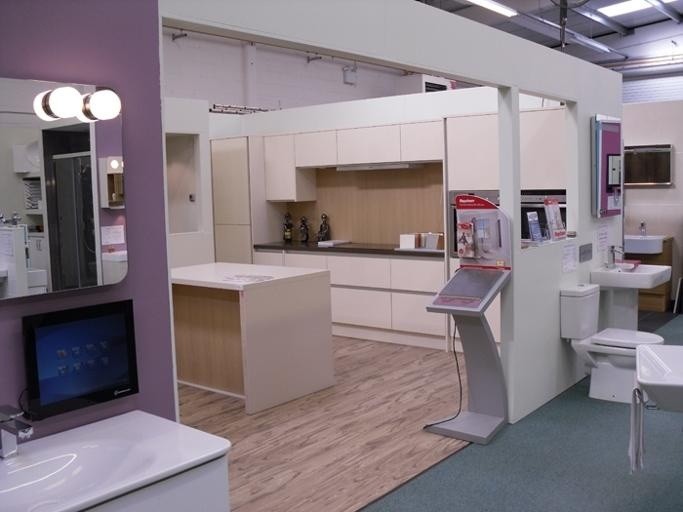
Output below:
[0,442,156,512]
[624,235,663,254]
[590,263,672,290]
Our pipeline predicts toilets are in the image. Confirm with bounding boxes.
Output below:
[560,284,664,403]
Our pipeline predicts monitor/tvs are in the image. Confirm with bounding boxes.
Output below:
[23,298,139,418]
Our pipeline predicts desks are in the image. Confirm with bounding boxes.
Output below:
[169,262,337,415]
[0,408,231,510]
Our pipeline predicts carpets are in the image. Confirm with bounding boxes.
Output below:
[349,308,681,511]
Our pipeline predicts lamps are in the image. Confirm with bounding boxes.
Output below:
[31,85,123,125]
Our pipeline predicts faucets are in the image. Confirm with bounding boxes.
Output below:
[607,246,624,268]
[640,222,647,236]
[0,405,33,458]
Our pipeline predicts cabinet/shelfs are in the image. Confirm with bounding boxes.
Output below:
[24,232,44,271]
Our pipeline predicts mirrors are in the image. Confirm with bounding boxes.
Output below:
[0,76,130,301]
[589,112,625,220]
